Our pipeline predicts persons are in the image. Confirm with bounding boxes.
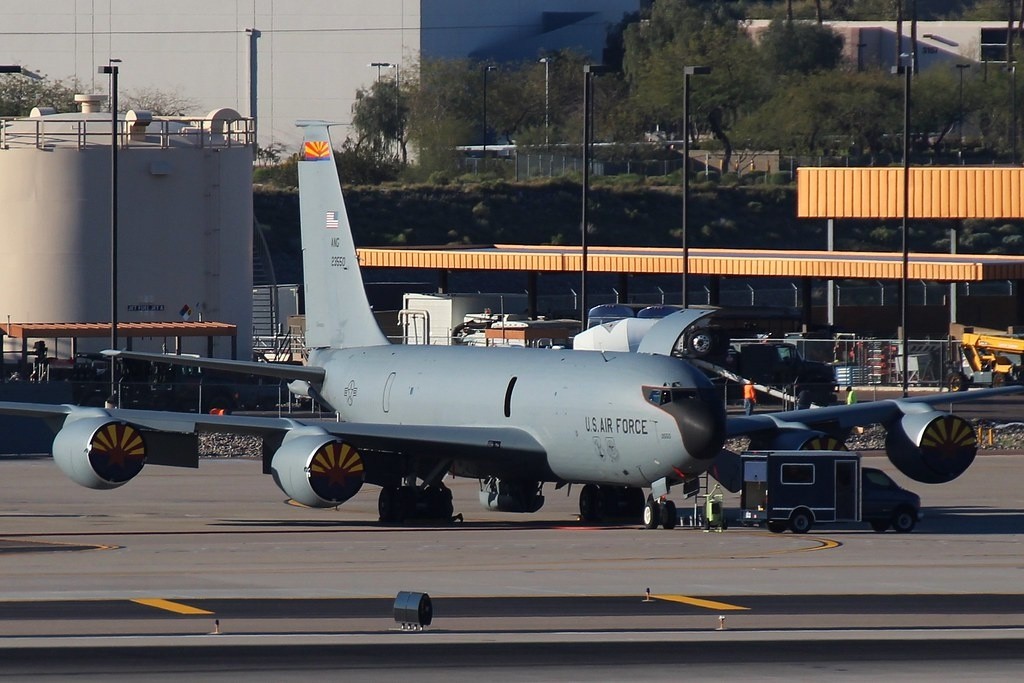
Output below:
[797,388,814,411]
[743,378,756,414]
[847,385,856,404]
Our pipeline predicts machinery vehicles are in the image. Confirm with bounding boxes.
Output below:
[486,314,583,349]
[453,308,527,348]
[946,332,1024,391]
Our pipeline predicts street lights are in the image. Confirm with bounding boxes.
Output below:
[385,62,405,159]
[99,57,133,394]
[891,50,916,402]
[953,61,971,105]
[483,61,496,145]
[580,63,596,334]
[564,280,800,309]
[369,61,391,156]
[539,54,552,145]
[673,62,714,306]
[833,279,1014,306]
[1003,64,1018,163]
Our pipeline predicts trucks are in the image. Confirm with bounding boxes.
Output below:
[396,288,530,347]
[252,281,304,350]
[738,449,922,534]
[682,328,837,404]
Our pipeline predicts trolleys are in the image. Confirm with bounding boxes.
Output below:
[701,484,728,530]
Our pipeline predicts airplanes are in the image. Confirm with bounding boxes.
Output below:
[0,114,1024,525]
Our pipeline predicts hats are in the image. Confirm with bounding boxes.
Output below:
[846,386,851,390]
